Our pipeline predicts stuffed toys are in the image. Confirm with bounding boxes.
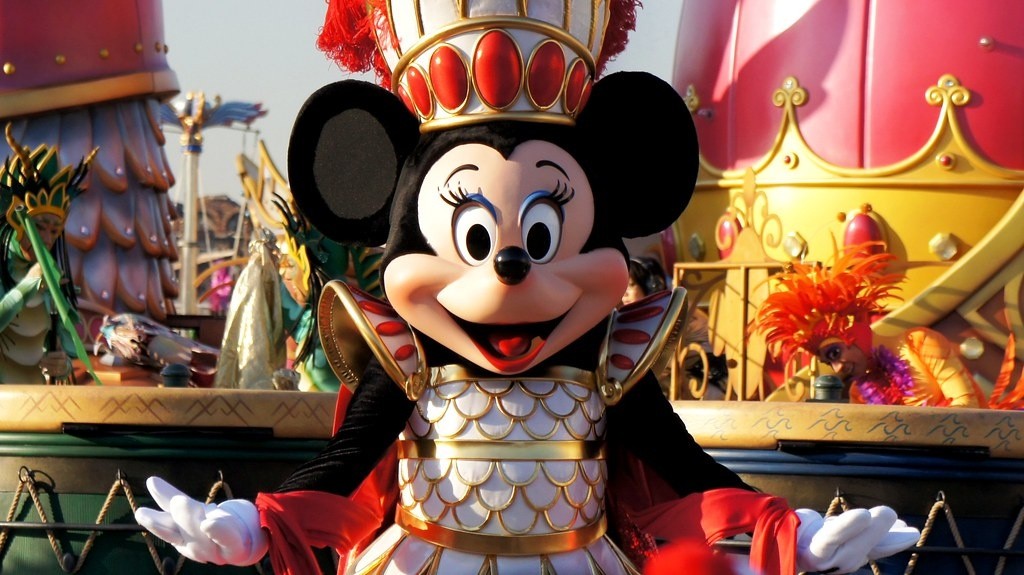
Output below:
[134,1,924,575]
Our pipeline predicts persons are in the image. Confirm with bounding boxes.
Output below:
[801,318,991,408]
[0,142,101,386]
[621,256,728,401]
[275,251,340,393]
